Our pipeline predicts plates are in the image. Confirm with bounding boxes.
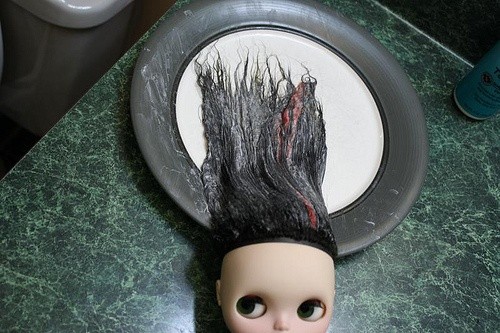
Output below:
[130,0,430,260]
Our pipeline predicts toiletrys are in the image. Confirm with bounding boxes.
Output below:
[452,32,500,122]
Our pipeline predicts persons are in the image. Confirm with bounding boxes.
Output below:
[193,37,338,333]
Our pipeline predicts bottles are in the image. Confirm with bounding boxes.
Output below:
[451,40,500,122]
